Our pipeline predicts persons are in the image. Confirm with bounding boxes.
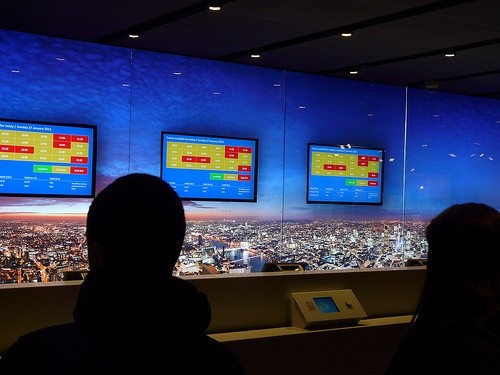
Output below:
[384,201,500,375]
[0,172,243,375]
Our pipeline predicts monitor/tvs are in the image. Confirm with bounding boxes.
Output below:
[1,119,97,200]
[159,129,258,203]
[306,143,384,206]
